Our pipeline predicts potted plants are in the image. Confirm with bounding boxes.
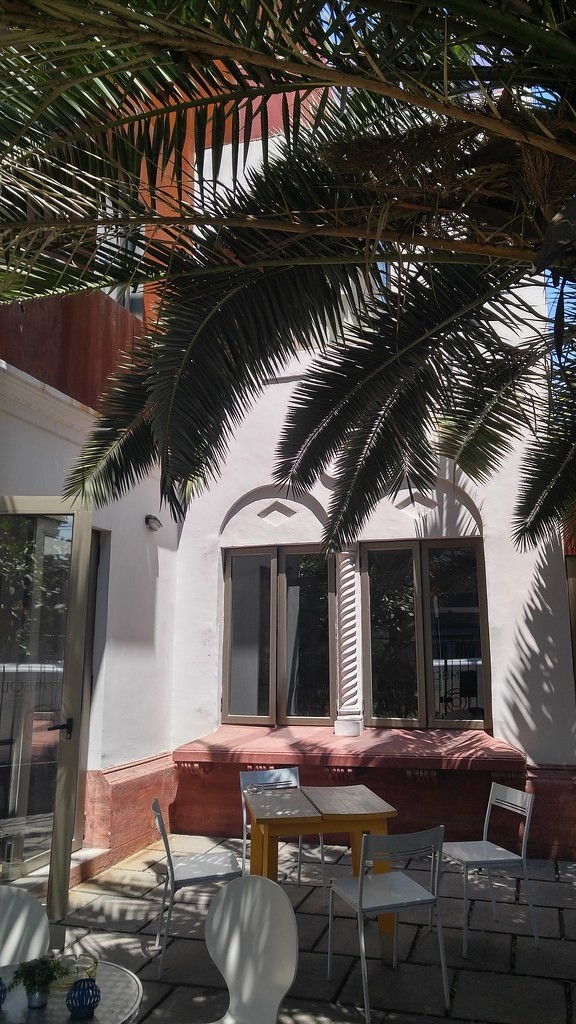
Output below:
[6,950,72,1008]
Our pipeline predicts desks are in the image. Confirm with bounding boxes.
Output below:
[241,783,399,969]
[0,960,144,1024]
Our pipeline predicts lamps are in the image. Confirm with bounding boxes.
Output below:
[146,514,164,531]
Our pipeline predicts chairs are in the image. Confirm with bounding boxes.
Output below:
[326,824,451,1024]
[239,765,303,887]
[435,781,541,951]
[150,797,243,980]
[0,885,51,966]
[205,874,299,1024]
